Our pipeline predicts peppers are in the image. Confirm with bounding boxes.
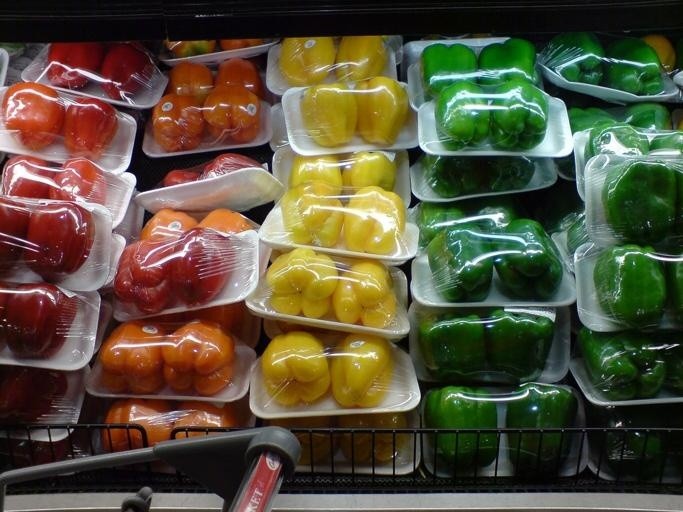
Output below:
[0,30,683,481]
